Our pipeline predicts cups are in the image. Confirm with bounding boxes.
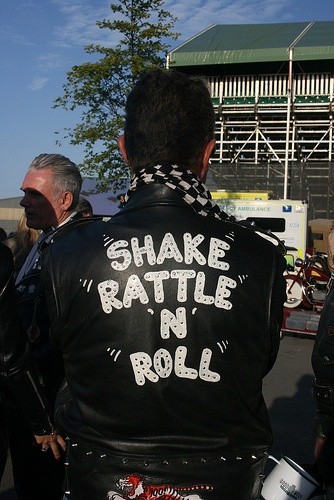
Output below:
[260,454,320,500]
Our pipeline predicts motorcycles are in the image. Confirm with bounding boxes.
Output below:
[280,246,334,312]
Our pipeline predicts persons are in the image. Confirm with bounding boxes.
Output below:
[312,228,334,500]
[0,154,93,500]
[19,69,289,500]
